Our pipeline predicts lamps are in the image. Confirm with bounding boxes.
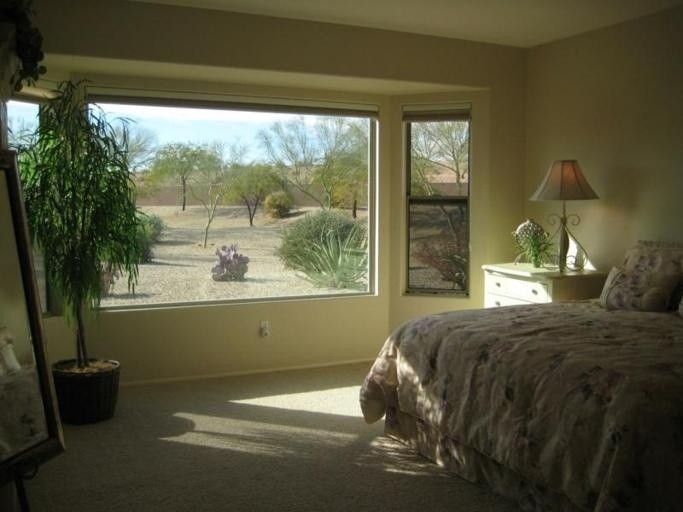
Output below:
[528,159,600,273]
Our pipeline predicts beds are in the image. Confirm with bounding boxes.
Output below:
[358,297,683,512]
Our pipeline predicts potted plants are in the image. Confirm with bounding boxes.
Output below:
[3,74,156,426]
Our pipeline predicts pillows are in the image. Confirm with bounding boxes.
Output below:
[592,241,683,313]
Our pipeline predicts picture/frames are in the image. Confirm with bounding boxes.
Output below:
[0,149,67,486]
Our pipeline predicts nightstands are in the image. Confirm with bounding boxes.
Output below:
[481,264,608,312]
